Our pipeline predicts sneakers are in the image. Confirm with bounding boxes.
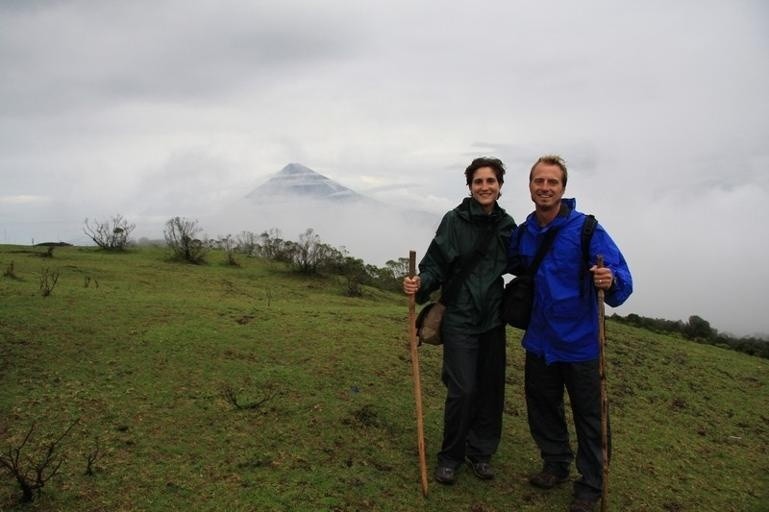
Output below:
[436,467,456,484]
[571,495,599,512]
[465,455,494,479]
[530,472,569,487]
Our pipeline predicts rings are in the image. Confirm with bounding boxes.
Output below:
[598,278,602,284]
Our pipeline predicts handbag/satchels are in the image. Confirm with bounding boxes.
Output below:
[499,275,535,329]
[415,303,447,347]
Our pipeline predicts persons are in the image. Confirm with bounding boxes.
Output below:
[501,153,636,510]
[402,155,522,485]
